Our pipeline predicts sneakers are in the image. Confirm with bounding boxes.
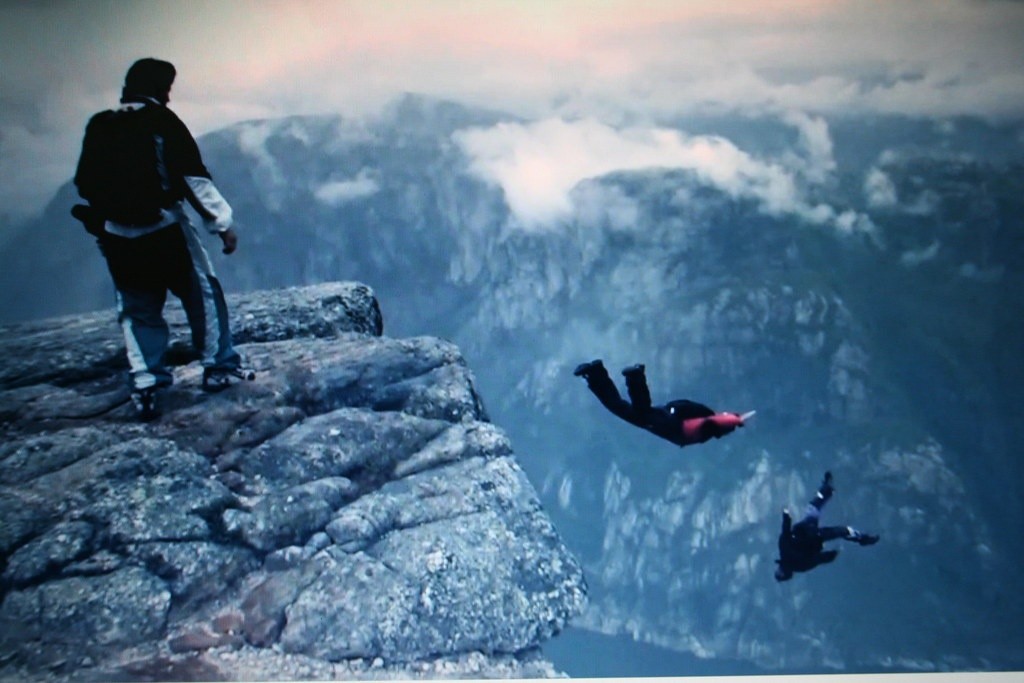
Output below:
[574,359,602,376]
[131,391,157,423]
[622,363,645,376]
[203,367,255,393]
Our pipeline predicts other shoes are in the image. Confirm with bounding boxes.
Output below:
[868,533,881,544]
[825,469,836,492]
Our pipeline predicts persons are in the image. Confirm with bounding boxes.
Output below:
[773,469,881,582]
[69,57,256,418]
[574,358,756,447]
